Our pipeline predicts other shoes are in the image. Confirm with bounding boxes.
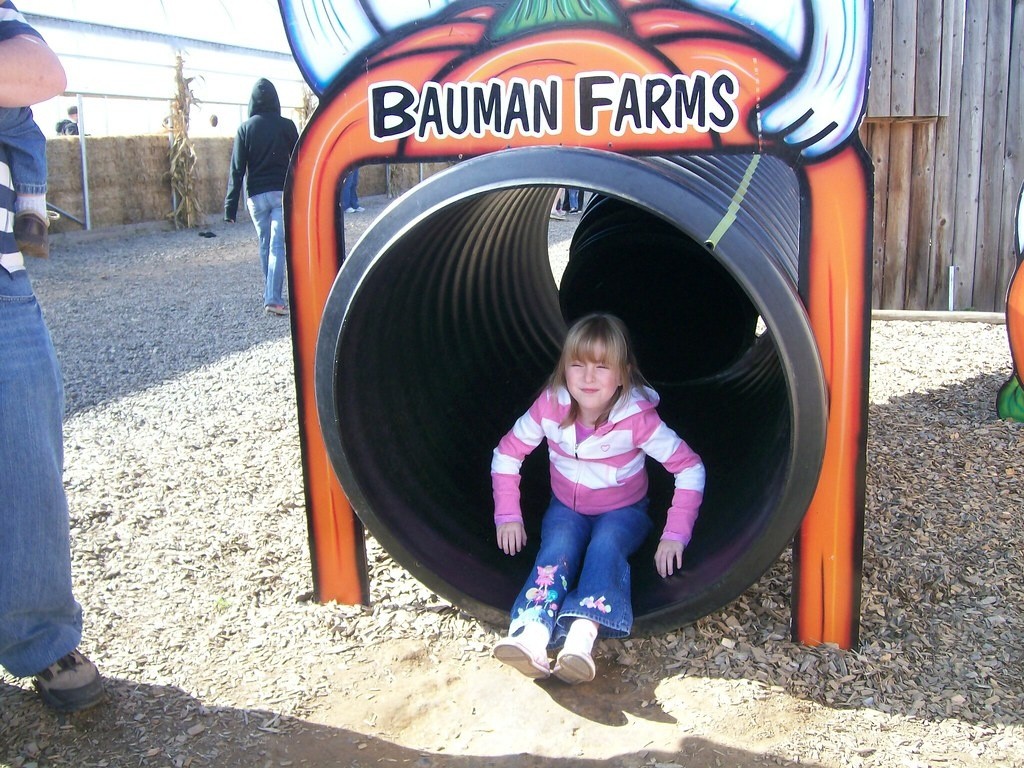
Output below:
[14,210,54,260]
[345,206,355,214]
[548,647,597,687]
[492,637,551,681]
[550,208,584,220]
[356,206,366,213]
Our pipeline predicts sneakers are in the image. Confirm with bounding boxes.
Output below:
[28,647,105,712]
[264,300,292,316]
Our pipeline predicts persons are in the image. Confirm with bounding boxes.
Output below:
[494,312,705,686]
[225,79,300,315]
[556,189,584,215]
[0,0,105,715]
[341,168,365,214]
